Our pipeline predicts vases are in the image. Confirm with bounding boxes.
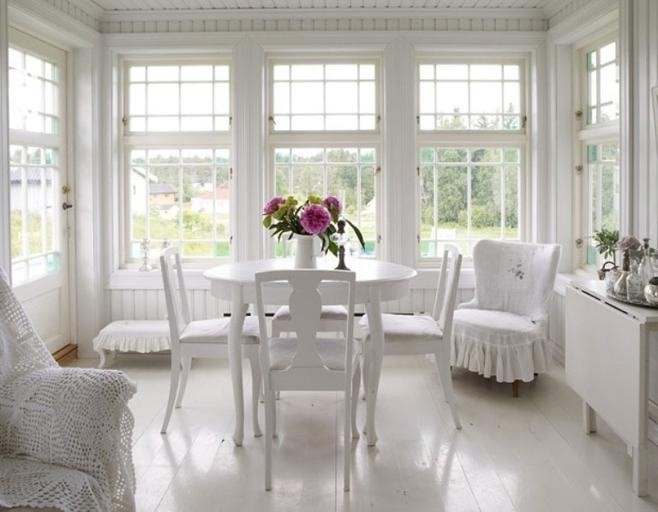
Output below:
[291,233,316,268]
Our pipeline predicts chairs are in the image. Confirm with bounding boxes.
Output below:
[359,249,464,434]
[252,268,361,492]
[424,237,562,399]
[0,265,141,511]
[159,244,267,438]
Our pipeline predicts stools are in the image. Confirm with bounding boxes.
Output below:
[92,319,186,370]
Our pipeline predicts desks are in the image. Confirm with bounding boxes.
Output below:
[563,279,658,497]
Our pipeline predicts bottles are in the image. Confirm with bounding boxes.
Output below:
[604,238,658,304]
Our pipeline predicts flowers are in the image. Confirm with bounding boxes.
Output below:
[619,236,640,252]
[261,192,367,258]
